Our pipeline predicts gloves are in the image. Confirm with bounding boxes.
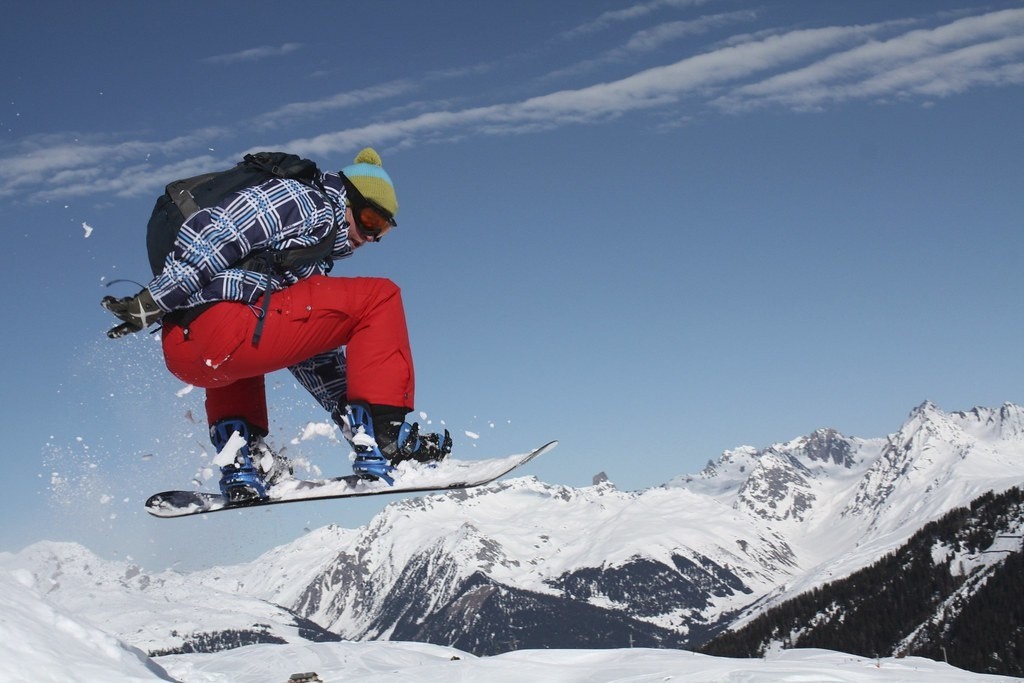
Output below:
[101,288,166,339]
[332,408,354,446]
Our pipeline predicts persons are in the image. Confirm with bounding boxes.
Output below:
[101,146,453,505]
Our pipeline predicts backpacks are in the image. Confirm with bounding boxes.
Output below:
[145,152,338,278]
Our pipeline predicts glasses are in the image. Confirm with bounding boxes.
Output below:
[350,197,396,243]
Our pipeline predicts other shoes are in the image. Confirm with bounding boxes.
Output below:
[214,418,293,505]
[338,400,452,478]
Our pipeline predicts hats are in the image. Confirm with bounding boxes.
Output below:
[338,147,398,218]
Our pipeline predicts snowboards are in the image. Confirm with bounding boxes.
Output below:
[144,437,560,519]
